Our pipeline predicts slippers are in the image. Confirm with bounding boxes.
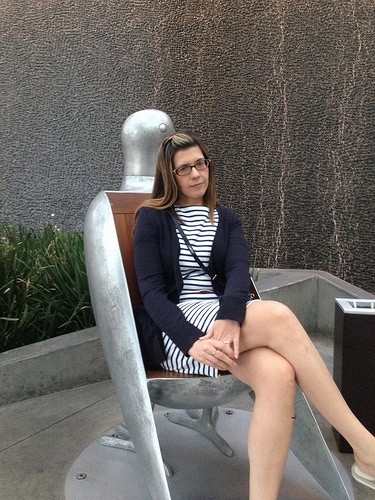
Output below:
[352,462,375,490]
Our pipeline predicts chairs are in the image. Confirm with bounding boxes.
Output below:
[82,191,357,500]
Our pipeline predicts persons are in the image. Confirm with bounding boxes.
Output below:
[134,134,375,500]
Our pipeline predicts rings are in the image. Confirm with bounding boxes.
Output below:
[212,351,216,356]
[224,341,230,343]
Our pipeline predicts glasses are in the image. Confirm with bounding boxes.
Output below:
[171,158,211,176]
